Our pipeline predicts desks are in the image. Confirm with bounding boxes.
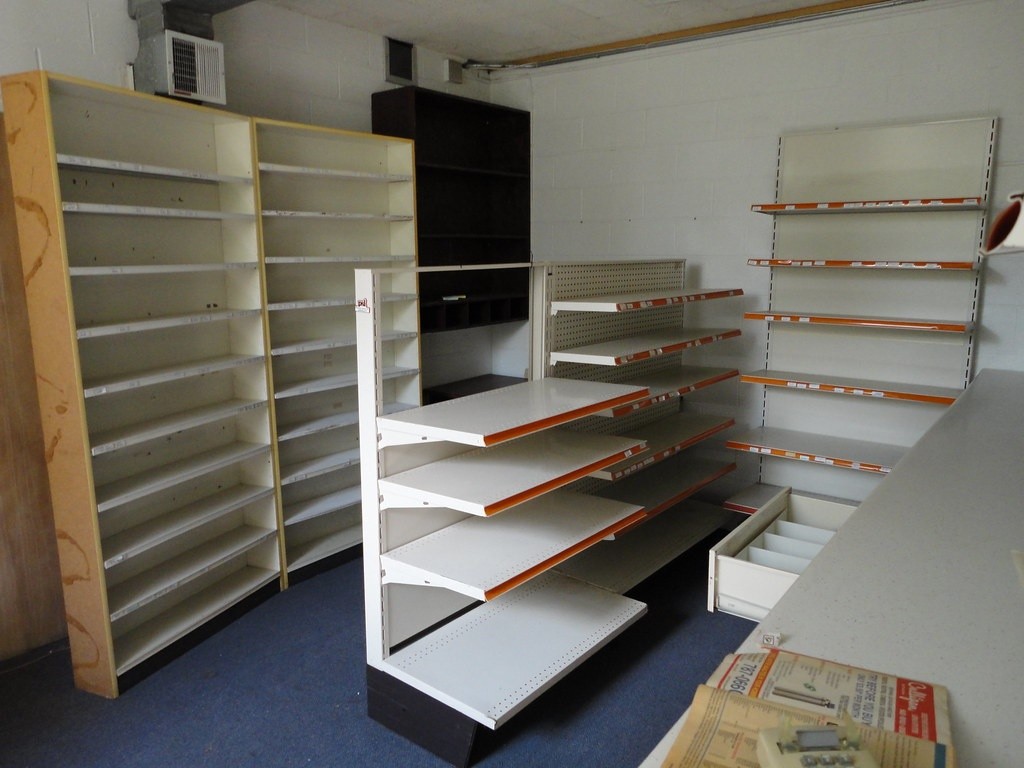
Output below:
[628,369,1024,768]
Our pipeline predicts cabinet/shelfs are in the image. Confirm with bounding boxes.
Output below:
[252,113,426,599]
[371,84,533,330]
[707,114,998,518]
[703,483,864,624]
[0,72,286,703]
[350,263,650,768]
[535,257,741,599]
